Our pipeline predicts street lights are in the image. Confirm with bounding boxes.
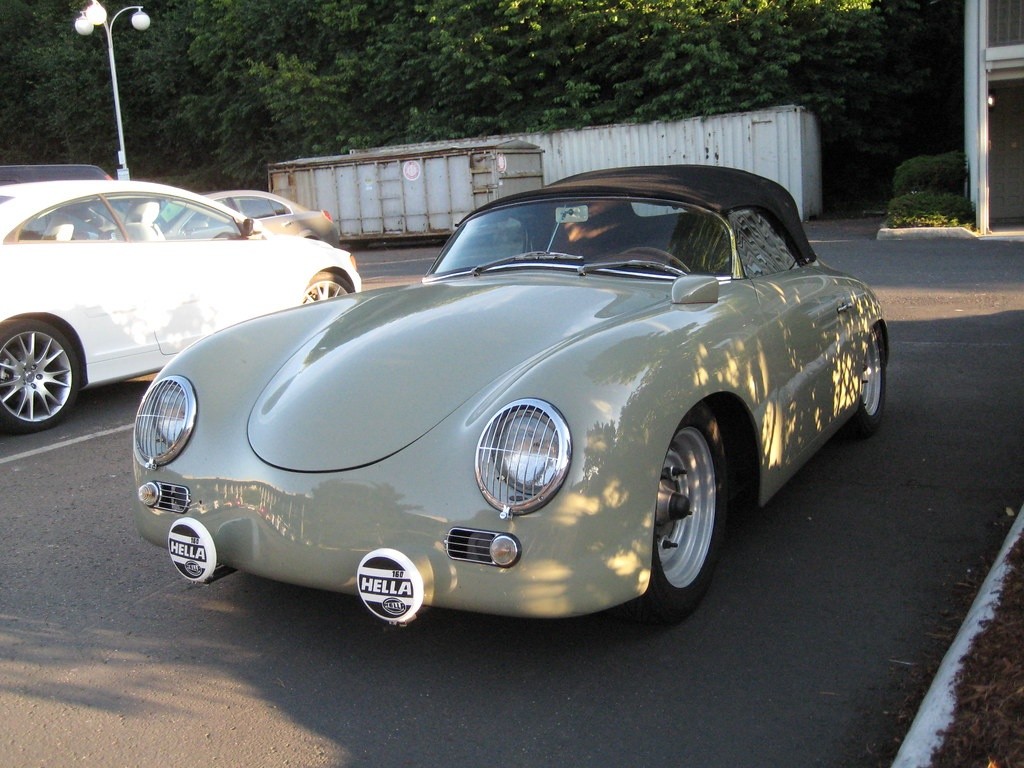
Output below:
[74,0,150,182]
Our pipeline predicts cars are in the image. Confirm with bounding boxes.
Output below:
[1,163,364,434]
[133,162,893,629]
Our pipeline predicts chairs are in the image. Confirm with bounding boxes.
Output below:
[671,211,731,275]
[117,202,166,242]
[48,223,74,241]
